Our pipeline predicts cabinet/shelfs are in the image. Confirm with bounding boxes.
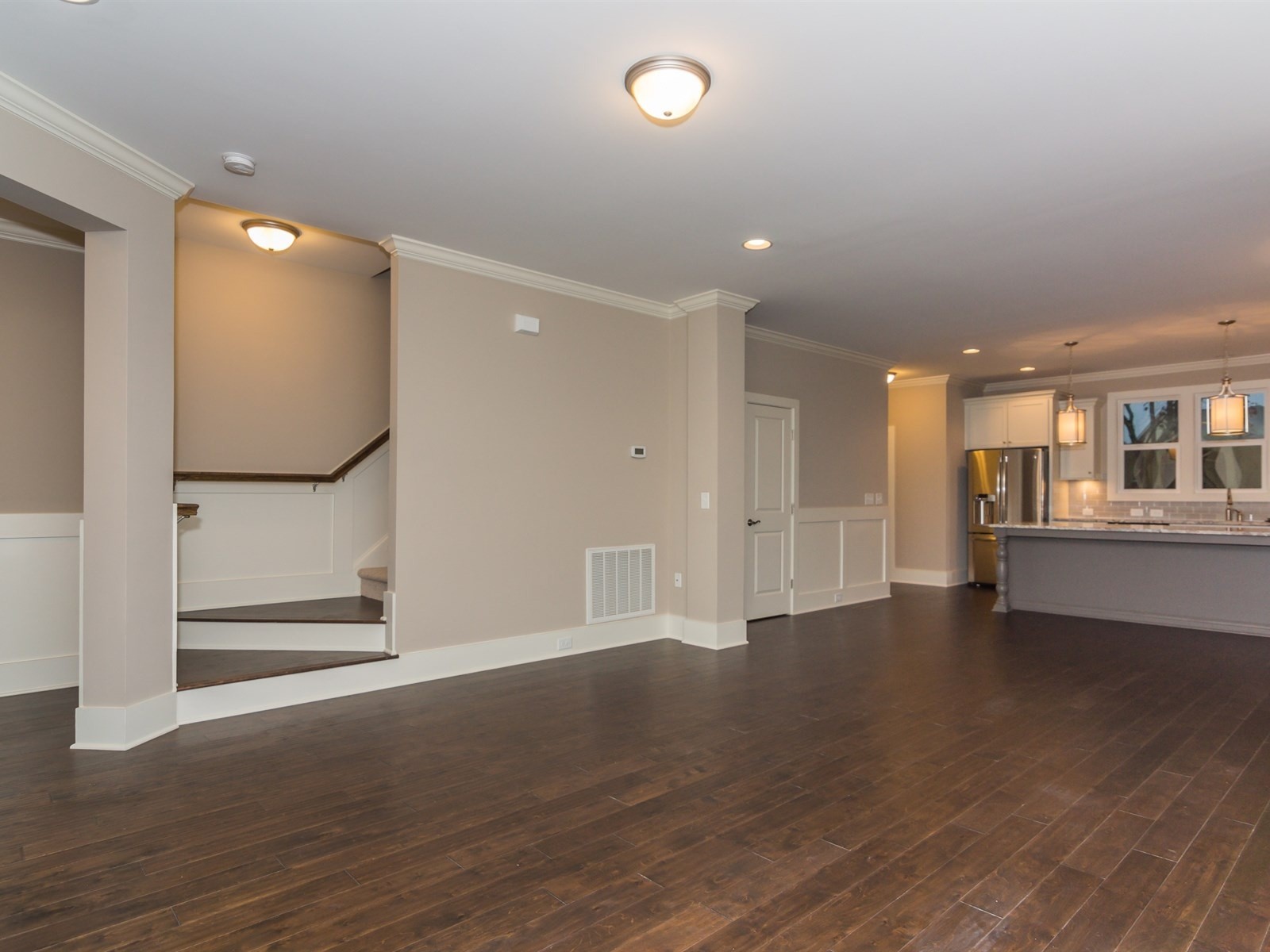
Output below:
[1058,398,1104,480]
[963,389,1070,451]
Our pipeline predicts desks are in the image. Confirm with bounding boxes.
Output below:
[986,520,1270,639]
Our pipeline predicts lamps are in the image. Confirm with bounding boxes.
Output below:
[241,219,299,252]
[1057,342,1086,444]
[624,54,711,120]
[1206,319,1249,436]
